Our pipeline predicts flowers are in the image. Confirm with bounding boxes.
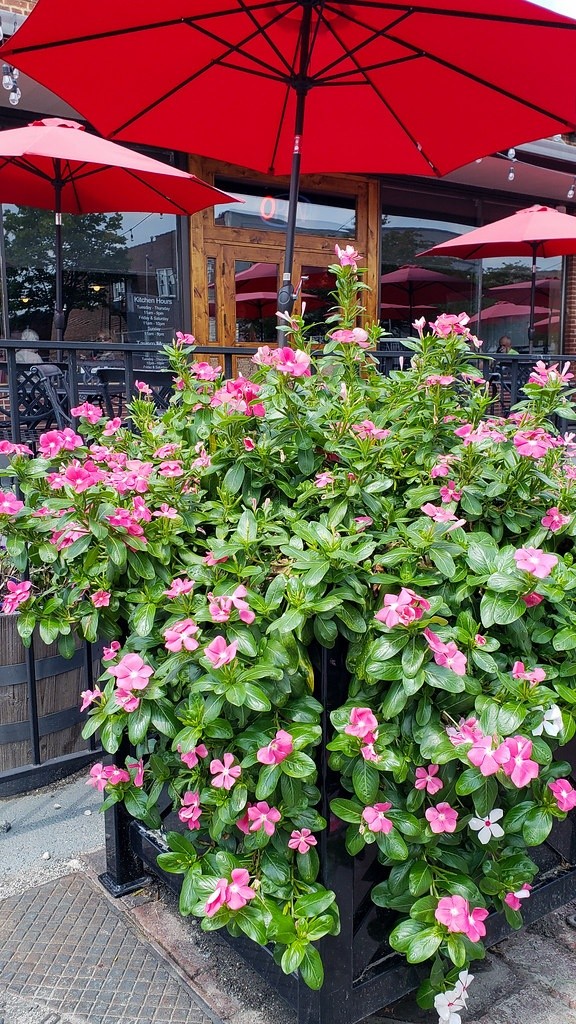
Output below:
[0,247,575,1024]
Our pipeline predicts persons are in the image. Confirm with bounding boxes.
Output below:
[497,337,519,354]
[93,328,123,359]
[15,329,44,384]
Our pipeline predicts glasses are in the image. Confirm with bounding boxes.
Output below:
[97,336,112,342]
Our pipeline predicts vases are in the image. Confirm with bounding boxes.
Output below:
[99,629,576,1024]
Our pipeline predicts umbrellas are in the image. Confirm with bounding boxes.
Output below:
[0,0,576,349]
[378,265,476,336]
[415,202,576,345]
[208,263,338,341]
[463,279,561,354]
[0,119,246,360]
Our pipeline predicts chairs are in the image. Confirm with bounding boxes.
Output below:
[1,360,179,431]
